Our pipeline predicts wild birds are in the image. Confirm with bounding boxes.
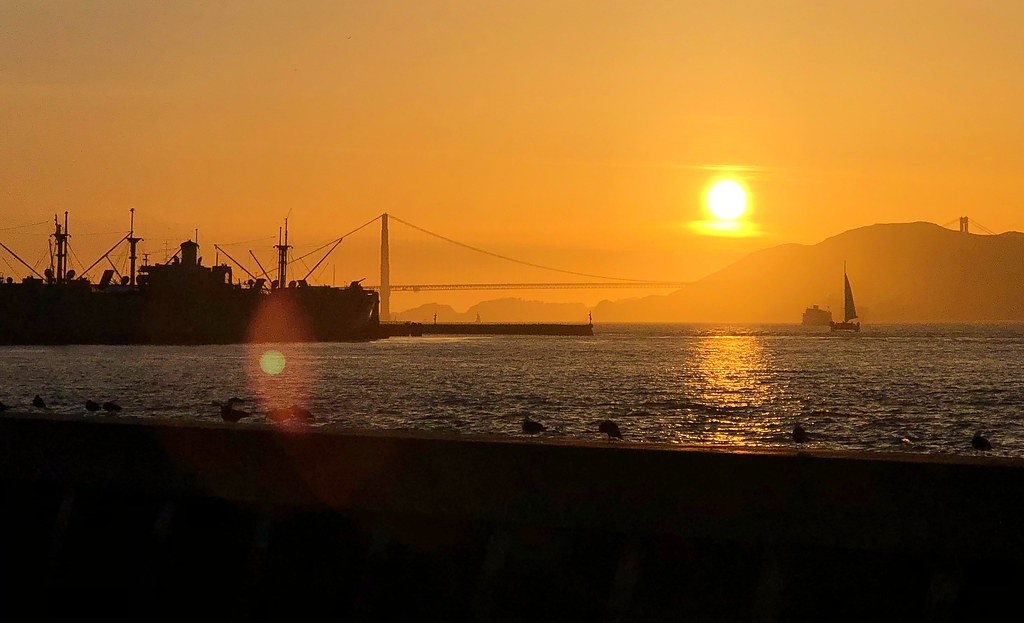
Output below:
[793,422,807,449]
[594,419,625,442]
[522,416,547,437]
[0,394,46,411]
[216,396,314,427]
[972,430,991,456]
[86,398,124,415]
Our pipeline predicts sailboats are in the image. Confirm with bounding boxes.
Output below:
[829,259,861,330]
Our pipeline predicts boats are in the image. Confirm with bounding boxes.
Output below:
[802,305,835,326]
[0,207,381,344]
